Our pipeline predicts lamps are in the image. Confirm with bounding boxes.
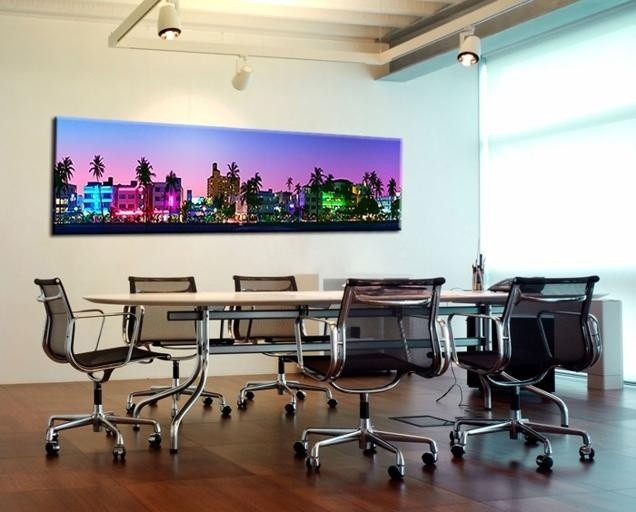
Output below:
[454,24,482,68]
[155,1,185,43]
[231,54,254,92]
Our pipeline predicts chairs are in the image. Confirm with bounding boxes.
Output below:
[228,275,339,412]
[33,275,171,461]
[448,275,604,469]
[294,277,450,479]
[123,274,233,431]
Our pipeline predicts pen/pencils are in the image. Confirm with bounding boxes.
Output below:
[472,254,485,291]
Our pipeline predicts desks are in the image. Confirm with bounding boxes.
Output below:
[82,291,615,453]
